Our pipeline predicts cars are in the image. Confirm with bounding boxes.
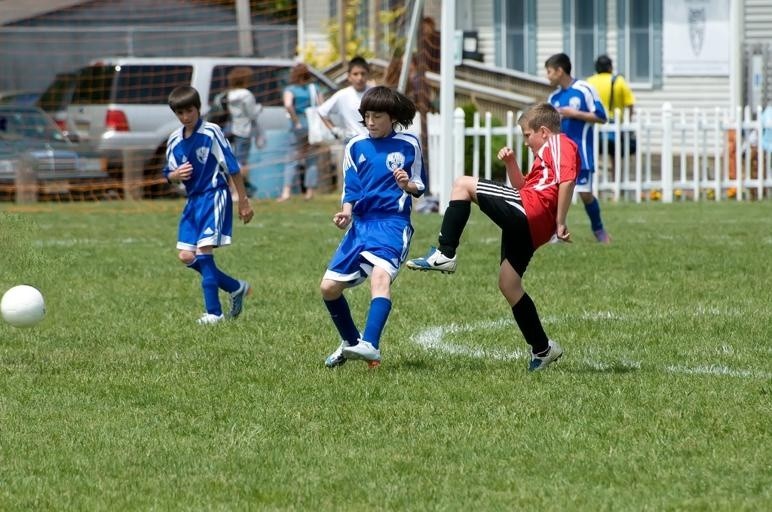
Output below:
[1,107,80,191]
[1,90,48,106]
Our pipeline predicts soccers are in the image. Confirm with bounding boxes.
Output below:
[0,285,45,327]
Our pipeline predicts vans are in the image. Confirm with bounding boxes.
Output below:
[38,69,80,151]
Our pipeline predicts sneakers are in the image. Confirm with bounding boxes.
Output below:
[528,340,563,374]
[406,247,457,274]
[196,313,226,325]
[227,280,250,318]
[343,338,381,362]
[594,227,609,244]
[325,332,363,368]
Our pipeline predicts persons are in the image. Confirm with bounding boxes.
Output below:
[585,55,637,195]
[544,52,613,245]
[276,62,324,202]
[422,16,440,75]
[319,86,426,367]
[222,67,266,202]
[404,101,581,372]
[315,56,372,147]
[161,85,254,326]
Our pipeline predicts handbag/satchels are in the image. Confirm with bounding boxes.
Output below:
[206,109,231,124]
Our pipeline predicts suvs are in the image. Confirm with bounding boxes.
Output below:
[66,57,338,194]
